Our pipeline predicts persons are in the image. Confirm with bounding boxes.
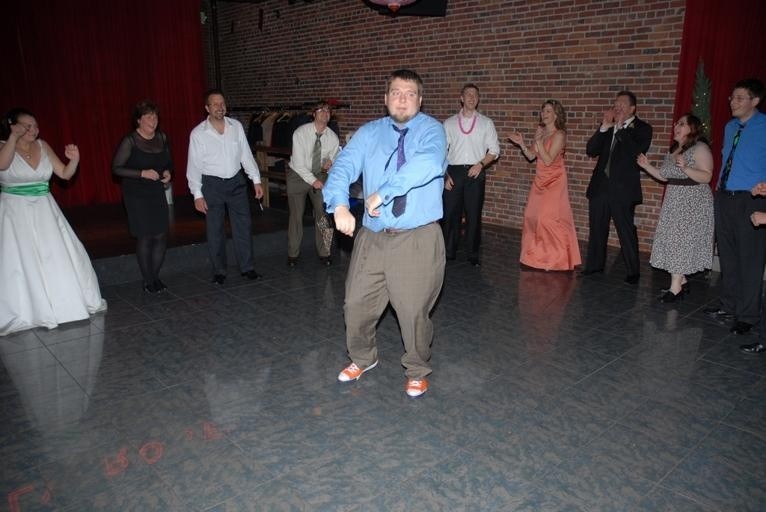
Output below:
[0,111,107,336]
[438,82,499,268]
[111,103,177,293]
[285,100,340,268]
[322,70,446,396]
[506,99,581,273]
[186,91,263,285]
[711,81,765,354]
[585,89,654,273]
[637,110,716,304]
[747,180,765,228]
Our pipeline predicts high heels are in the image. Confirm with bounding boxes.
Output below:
[658,287,684,303]
[661,282,689,294]
[153,278,167,292]
[143,281,161,295]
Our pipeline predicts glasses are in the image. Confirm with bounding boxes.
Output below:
[726,94,751,101]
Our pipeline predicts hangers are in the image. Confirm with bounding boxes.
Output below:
[252,101,332,124]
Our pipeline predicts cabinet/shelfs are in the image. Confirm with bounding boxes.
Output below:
[221,102,350,219]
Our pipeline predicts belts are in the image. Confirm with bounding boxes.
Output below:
[724,189,744,196]
[383,227,406,234]
[212,177,237,181]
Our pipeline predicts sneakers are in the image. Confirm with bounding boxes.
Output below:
[406,376,428,398]
[337,358,379,382]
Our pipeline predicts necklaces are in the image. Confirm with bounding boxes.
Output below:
[457,115,476,134]
[14,142,37,159]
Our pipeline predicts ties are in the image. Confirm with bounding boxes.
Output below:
[390,123,409,218]
[605,122,627,178]
[313,132,322,190]
[719,125,745,190]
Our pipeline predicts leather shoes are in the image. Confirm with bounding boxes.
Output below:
[729,321,754,335]
[320,256,332,267]
[703,308,728,315]
[577,268,603,277]
[469,258,481,267]
[288,257,298,267]
[716,313,737,323]
[739,342,766,355]
[211,273,226,285]
[623,275,641,287]
[241,269,263,282]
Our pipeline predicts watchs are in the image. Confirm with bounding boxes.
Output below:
[479,160,485,169]
